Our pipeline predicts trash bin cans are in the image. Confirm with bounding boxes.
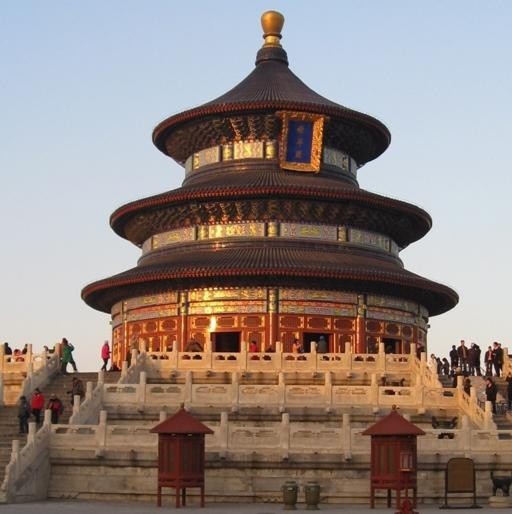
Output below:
[280,480,300,510]
[304,480,320,511]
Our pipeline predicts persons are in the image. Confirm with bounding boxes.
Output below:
[66,377,84,407]
[100,340,112,371]
[17,395,31,434]
[31,388,44,423]
[417,338,512,415]
[4,342,27,362]
[292,339,302,354]
[62,340,77,371]
[316,335,328,353]
[250,340,259,360]
[46,393,64,424]
